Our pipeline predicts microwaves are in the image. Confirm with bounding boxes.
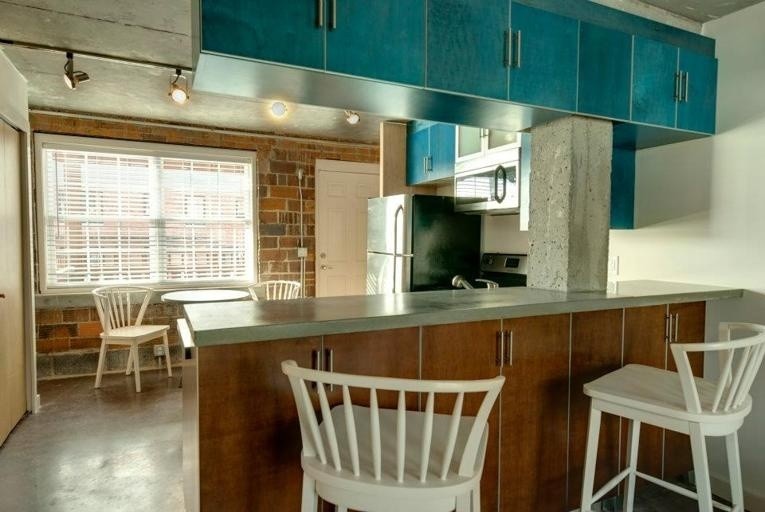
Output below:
[454,160,519,215]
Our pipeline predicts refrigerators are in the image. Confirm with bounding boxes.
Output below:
[366,193,480,295]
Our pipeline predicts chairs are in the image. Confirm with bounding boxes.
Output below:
[281,358,505,512]
[248,280,301,301]
[582,323,765,511]
[92,284,173,393]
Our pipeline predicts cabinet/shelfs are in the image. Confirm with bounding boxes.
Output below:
[614,14,717,150]
[455,124,519,174]
[407,120,455,187]
[192,1,427,120]
[576,1,632,122]
[177,305,421,512]
[620,301,705,498]
[426,1,576,131]
[421,312,569,512]
[566,310,622,511]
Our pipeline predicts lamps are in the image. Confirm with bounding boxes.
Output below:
[267,100,288,116]
[63,51,90,90]
[342,108,360,124]
[295,168,306,298]
[167,68,190,105]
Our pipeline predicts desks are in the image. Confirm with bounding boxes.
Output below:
[162,289,250,303]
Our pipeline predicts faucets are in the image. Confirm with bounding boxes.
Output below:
[450,275,498,290]
